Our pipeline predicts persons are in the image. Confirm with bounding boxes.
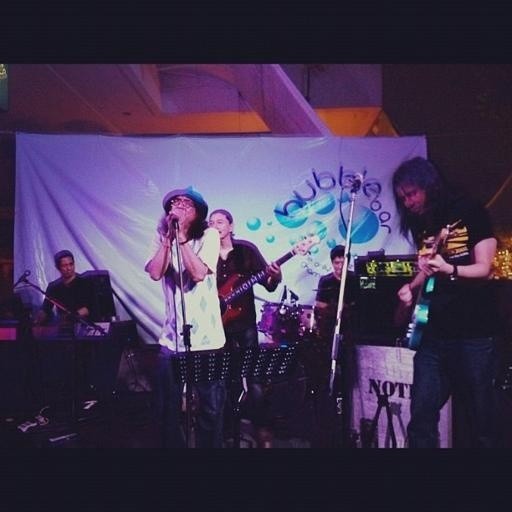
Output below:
[314,243,361,316]
[34,248,90,325]
[143,185,227,449]
[391,156,498,450]
[208,209,282,423]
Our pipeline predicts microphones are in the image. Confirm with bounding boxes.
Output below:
[15,270,31,287]
[172,218,179,230]
[352,173,363,194]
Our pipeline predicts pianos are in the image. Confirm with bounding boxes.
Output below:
[2,309,117,422]
[319,249,419,350]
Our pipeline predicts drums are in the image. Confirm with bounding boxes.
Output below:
[290,304,316,339]
[256,300,291,334]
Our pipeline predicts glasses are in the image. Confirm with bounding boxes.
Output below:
[170,198,195,207]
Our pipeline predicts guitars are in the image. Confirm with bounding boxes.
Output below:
[218,235,321,325]
[395,217,464,348]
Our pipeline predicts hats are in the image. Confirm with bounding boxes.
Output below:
[163,186,208,220]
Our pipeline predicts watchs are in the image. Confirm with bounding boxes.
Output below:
[450,263,459,282]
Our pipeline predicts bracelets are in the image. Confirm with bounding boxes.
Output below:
[178,239,189,245]
[271,279,282,285]
